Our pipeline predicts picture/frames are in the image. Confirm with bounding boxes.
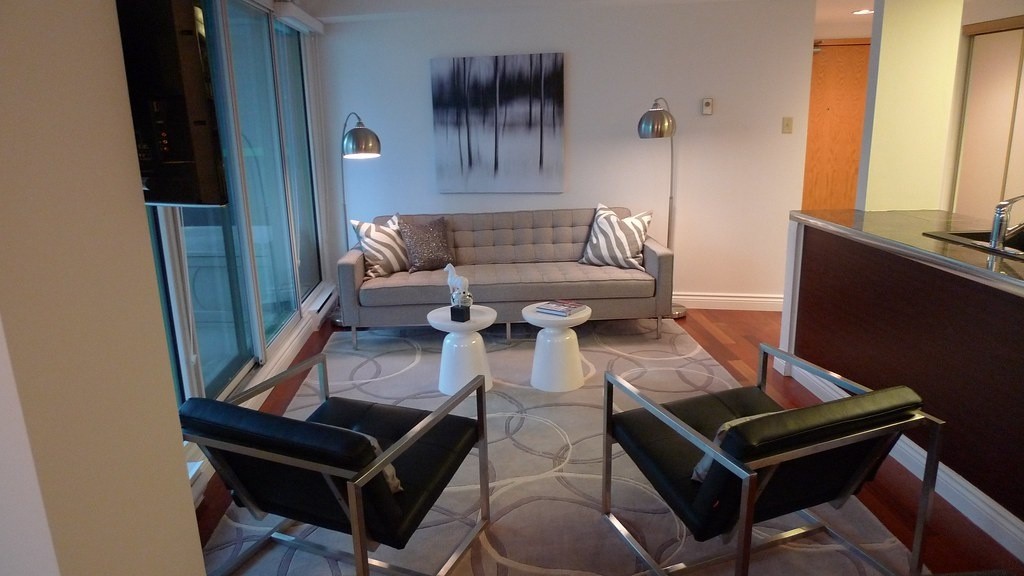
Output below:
[430,51,566,195]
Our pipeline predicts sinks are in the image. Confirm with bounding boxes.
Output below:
[922,226,1024,262]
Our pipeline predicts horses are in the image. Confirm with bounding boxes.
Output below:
[442,263,469,293]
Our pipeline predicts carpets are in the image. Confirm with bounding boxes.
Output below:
[206,319,936,576]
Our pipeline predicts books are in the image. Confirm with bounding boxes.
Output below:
[536,300,586,317]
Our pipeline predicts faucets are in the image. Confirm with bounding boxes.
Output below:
[989,195,1024,250]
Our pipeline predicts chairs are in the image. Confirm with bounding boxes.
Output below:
[596,343,946,573]
[180,354,492,576]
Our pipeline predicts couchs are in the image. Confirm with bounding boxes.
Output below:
[336,202,675,353]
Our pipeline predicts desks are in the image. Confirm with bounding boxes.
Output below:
[771,207,1024,565]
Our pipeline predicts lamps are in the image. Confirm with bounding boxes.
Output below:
[329,110,383,322]
[636,97,689,320]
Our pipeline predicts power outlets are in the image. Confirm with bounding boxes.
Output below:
[701,98,715,116]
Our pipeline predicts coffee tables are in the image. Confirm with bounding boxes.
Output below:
[426,305,499,399]
[521,297,593,393]
